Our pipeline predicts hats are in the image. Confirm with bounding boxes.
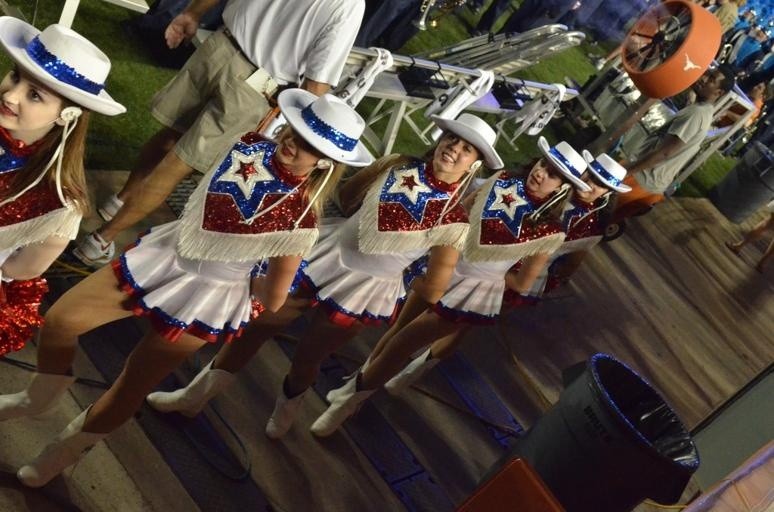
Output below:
[430,113,504,170]
[277,88,376,168]
[0,16,127,117]
[537,134,632,194]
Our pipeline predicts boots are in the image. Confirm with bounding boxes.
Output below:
[17,403,110,488]
[146,359,239,418]
[384,343,440,396]
[311,366,381,440]
[265,375,310,439]
[0,374,75,422]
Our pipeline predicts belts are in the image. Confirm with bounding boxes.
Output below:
[222,27,259,70]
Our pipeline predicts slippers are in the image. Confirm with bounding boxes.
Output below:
[724,241,739,256]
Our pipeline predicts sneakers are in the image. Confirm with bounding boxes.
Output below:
[97,193,124,222]
[73,231,116,267]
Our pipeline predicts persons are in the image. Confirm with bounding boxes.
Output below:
[145,112,505,441]
[692,0,774,160]
[471,0,645,41]
[558,66,734,285]
[72,0,366,268]
[308,134,632,438]
[0,15,127,357]
[0,88,374,488]
[726,213,774,273]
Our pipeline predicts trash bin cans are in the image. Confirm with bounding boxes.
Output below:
[476,352,699,512]
[708,141,774,224]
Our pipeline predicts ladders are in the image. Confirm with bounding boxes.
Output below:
[409,24,587,77]
